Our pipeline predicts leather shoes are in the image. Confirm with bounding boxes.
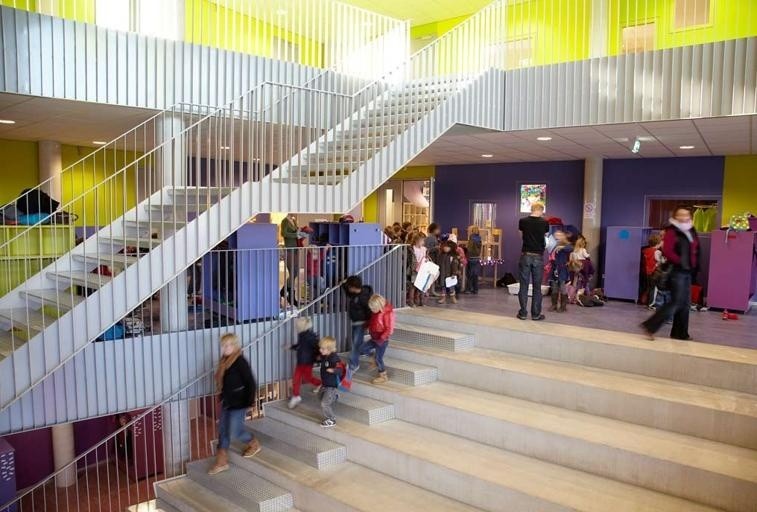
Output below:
[671,334,694,341]
[532,315,547,320]
[517,313,526,319]
[639,324,654,341]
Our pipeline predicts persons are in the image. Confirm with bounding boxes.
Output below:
[548,230,574,313]
[408,230,429,307]
[207,332,262,476]
[316,337,345,427]
[280,213,303,306]
[116,416,131,465]
[464,225,483,295]
[640,234,663,304]
[392,221,420,244]
[655,241,672,324]
[280,316,322,409]
[341,275,375,375]
[357,293,392,385]
[187,258,202,300]
[572,233,594,297]
[639,204,701,341]
[437,240,459,304]
[306,242,334,315]
[516,202,550,321]
[423,223,441,298]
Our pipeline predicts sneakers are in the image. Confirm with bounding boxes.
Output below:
[287,395,303,409]
[313,379,324,392]
[722,309,729,319]
[728,313,738,320]
[350,366,360,375]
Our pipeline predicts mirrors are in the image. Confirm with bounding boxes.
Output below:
[469,198,496,234]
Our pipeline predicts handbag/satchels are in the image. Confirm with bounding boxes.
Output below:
[416,255,433,271]
[50,211,78,223]
[2,219,19,225]
[17,188,58,212]
[445,276,458,287]
[0,204,22,218]
[653,228,676,289]
[19,213,50,225]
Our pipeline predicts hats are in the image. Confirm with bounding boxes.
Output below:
[403,221,412,229]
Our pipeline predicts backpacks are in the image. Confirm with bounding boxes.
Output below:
[569,251,584,273]
[334,360,353,393]
[378,308,396,335]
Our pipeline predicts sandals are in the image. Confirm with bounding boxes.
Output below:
[321,419,336,427]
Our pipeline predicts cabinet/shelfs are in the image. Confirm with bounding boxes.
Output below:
[705,229,755,313]
[402,202,430,234]
[603,227,643,304]
[0,224,76,358]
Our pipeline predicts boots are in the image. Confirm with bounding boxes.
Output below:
[287,290,298,307]
[438,294,446,304]
[242,432,262,458]
[548,294,558,311]
[419,292,427,307]
[280,287,288,299]
[408,289,416,307]
[449,292,457,304]
[557,294,568,313]
[208,448,230,475]
[372,370,389,384]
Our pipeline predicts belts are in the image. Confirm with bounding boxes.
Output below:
[522,252,543,256]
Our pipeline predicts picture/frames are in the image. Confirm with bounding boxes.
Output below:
[516,180,550,217]
